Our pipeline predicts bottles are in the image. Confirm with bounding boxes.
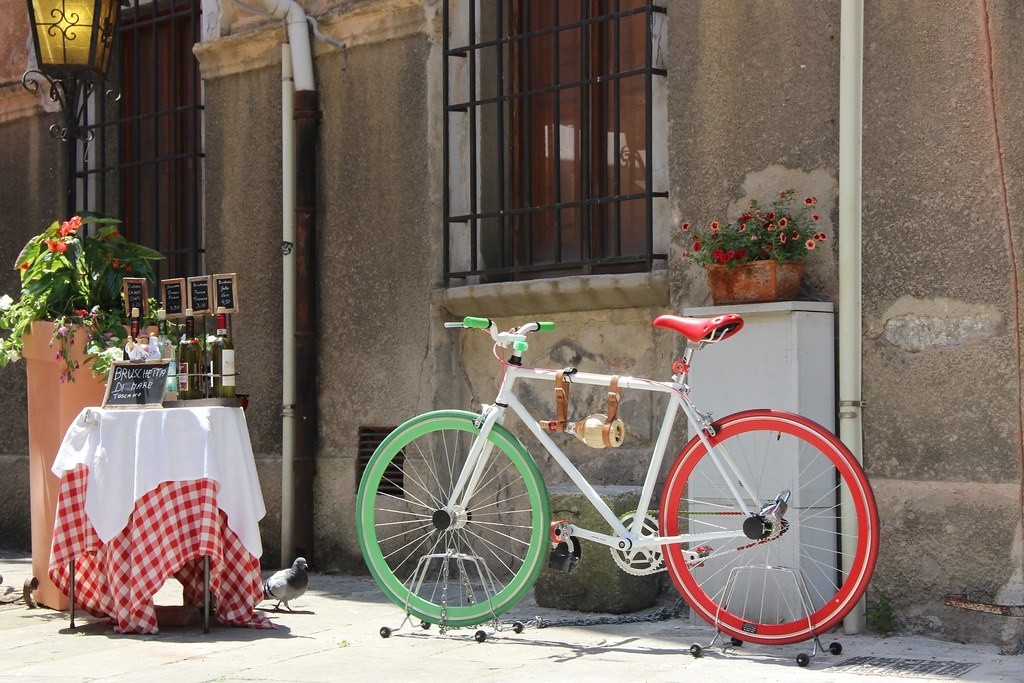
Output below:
[122,307,141,361]
[540,414,625,449]
[210,307,236,398]
[156,309,177,401]
[179,309,203,399]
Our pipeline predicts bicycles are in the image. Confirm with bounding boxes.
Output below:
[359,314,879,646]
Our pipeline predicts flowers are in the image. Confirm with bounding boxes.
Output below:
[0,209,217,390]
[671,186,827,271]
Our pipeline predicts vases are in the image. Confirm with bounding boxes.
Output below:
[706,260,807,308]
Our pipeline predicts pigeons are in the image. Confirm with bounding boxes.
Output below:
[262,556,309,613]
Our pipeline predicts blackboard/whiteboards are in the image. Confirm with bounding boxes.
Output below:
[101,360,172,409]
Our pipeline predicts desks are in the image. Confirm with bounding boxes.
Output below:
[47,400,278,636]
